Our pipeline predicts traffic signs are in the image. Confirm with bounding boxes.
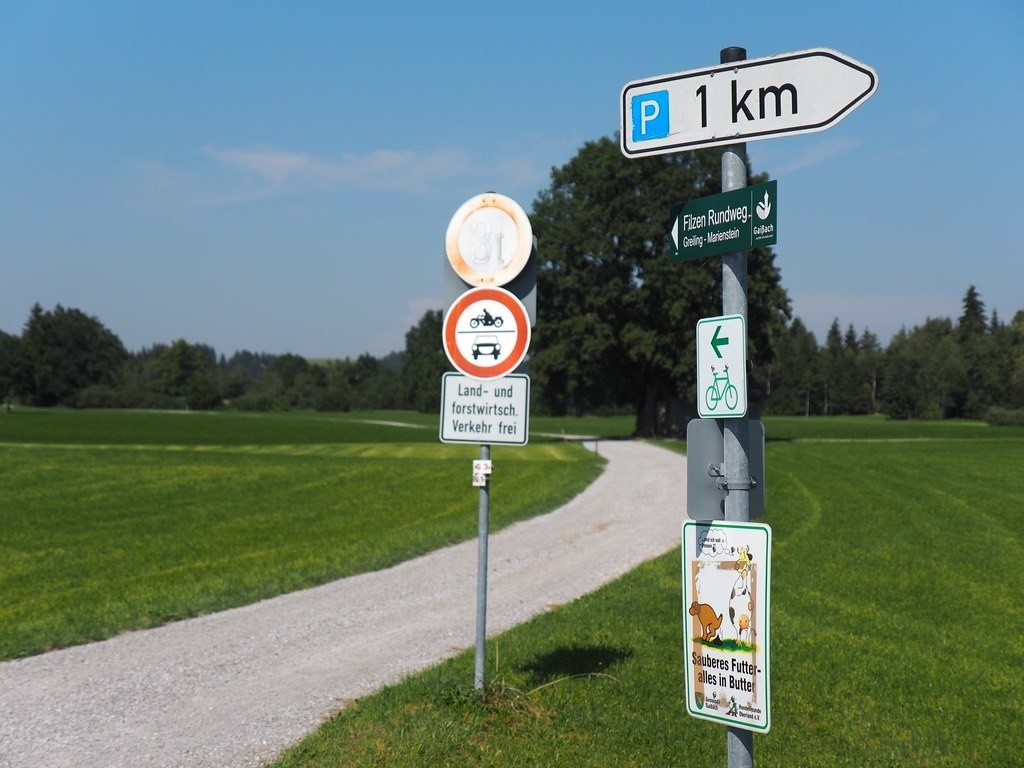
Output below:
[666,180,780,260]
